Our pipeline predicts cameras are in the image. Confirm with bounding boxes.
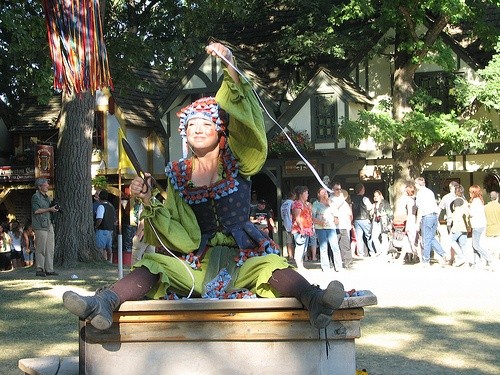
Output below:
[53,201,65,215]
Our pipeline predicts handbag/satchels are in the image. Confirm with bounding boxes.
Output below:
[296,234,306,246]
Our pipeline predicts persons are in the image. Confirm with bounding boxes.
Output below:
[30,178,61,277]
[279,182,389,271]
[249,198,277,236]
[90,189,156,268]
[63,42,346,329]
[387,177,500,272]
[0,219,35,271]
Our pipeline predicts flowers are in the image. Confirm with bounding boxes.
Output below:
[269,131,315,155]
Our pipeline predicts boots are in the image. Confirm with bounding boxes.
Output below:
[298,281,344,329]
[63,287,121,331]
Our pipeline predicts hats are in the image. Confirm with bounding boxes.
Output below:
[258,200,266,203]
[176,96,226,135]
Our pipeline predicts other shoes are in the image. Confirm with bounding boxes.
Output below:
[453,258,466,267]
[37,271,59,277]
